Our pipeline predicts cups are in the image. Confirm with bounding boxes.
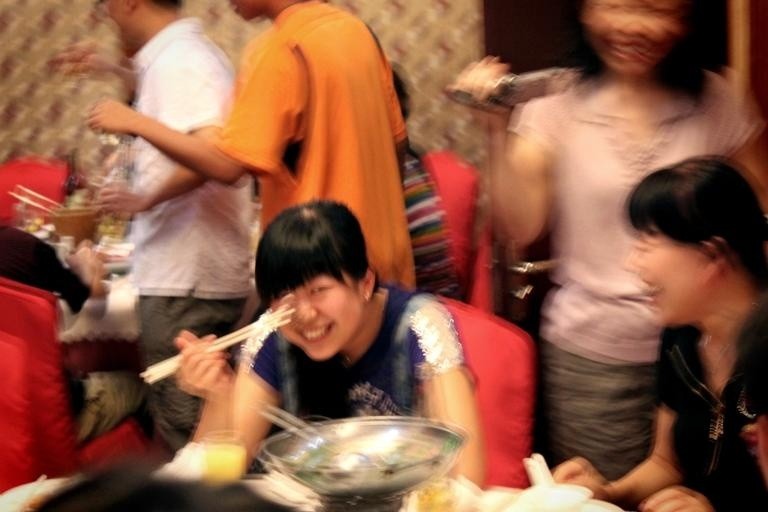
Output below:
[197,430,247,488]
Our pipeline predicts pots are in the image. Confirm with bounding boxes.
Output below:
[252,416,475,497]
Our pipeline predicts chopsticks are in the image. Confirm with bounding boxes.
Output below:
[139,308,297,386]
[265,405,323,446]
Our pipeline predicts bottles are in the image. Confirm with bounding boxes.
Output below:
[100,134,135,239]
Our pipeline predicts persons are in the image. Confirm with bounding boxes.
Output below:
[1,0,766,512]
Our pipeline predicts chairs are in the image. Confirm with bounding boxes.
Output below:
[0,151,537,512]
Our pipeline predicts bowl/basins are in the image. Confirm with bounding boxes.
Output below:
[516,481,593,512]
[52,207,97,244]
